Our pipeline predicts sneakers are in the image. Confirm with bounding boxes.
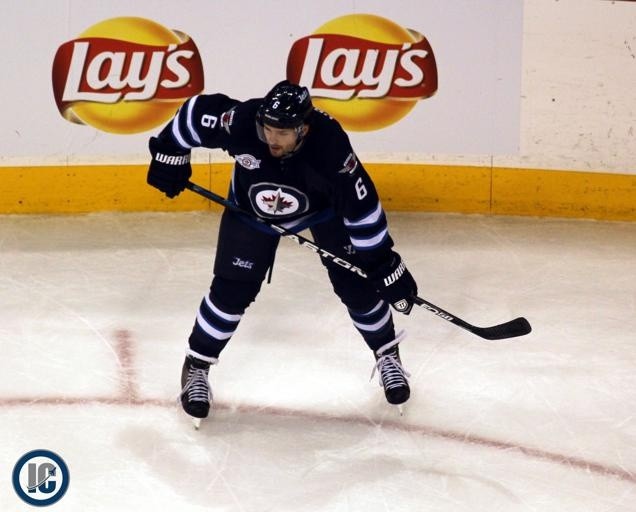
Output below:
[179,353,210,418]
[373,349,411,406]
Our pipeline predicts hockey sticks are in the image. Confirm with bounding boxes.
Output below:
[185,181,532,340]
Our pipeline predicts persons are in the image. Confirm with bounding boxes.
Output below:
[147,81,420,417]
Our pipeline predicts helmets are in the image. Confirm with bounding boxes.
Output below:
[255,80,311,130]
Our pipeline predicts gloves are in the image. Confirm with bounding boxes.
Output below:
[146,135,192,199]
[373,250,418,316]
